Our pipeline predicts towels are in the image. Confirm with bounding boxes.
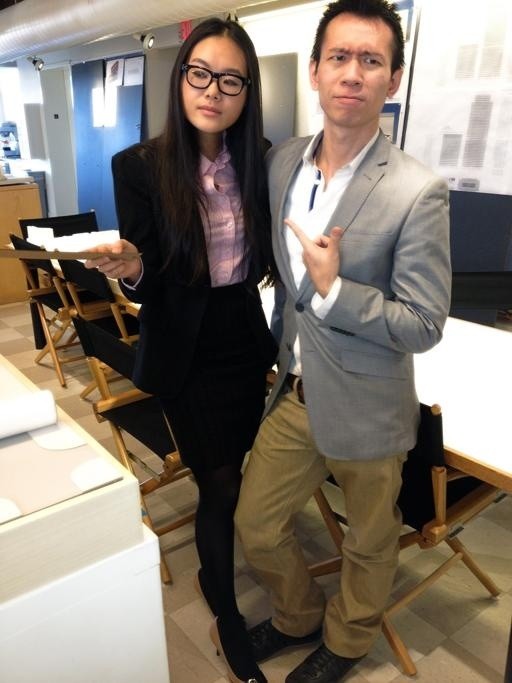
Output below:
[0,390,57,439]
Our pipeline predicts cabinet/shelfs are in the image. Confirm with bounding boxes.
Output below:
[1,178,47,304]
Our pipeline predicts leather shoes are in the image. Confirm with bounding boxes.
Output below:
[216,615,367,683]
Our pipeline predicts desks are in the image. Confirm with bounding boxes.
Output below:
[54,230,511,498]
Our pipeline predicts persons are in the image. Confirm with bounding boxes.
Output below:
[80,15,280,683]
[234,1,453,682]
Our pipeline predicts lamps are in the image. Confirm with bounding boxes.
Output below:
[133,33,155,49]
[26,55,44,71]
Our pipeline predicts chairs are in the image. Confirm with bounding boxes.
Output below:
[18,209,99,348]
[70,308,198,586]
[268,371,505,680]
[62,247,140,401]
[10,233,85,387]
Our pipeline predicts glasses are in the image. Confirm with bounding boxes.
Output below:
[181,64,251,97]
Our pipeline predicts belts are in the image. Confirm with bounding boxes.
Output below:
[286,373,305,405]
